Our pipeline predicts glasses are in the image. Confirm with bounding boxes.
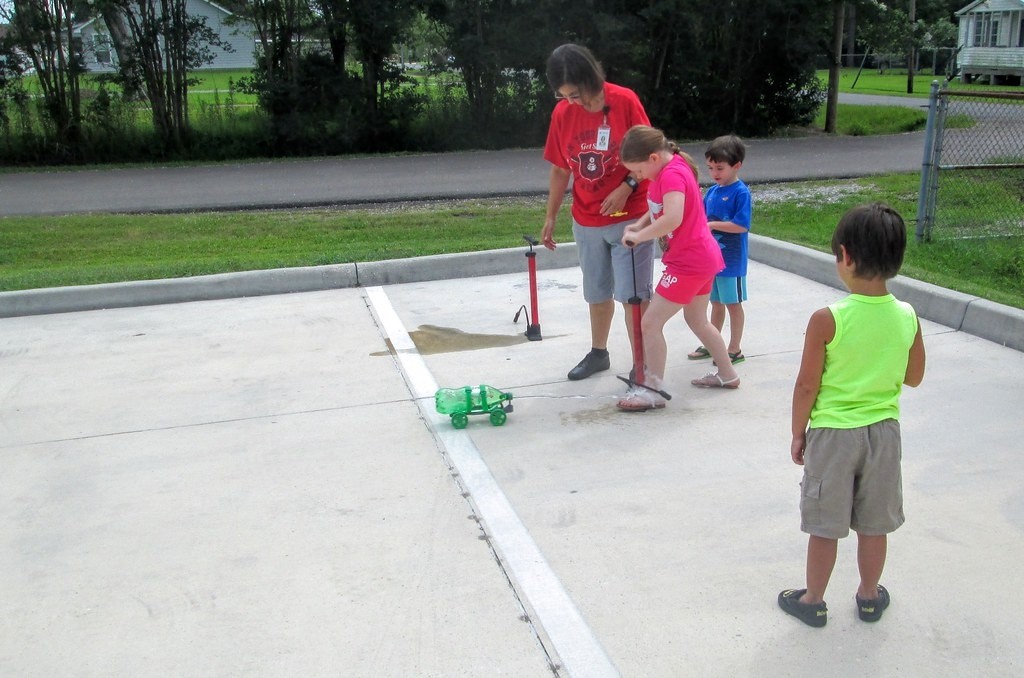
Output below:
[554,91,580,99]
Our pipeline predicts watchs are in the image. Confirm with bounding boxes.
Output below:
[624,175,638,192]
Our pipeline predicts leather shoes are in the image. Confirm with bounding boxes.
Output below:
[629,365,635,381]
[568,350,610,380]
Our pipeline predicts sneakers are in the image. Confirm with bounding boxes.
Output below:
[777,588,827,627]
[857,583,889,623]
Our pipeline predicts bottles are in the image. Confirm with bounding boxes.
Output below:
[436,385,513,414]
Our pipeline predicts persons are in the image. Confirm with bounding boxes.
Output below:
[540,44,654,388]
[616,124,741,410]
[777,202,926,627]
[687,135,752,366]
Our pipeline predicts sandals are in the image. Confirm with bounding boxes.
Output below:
[691,372,740,389]
[713,350,745,365]
[617,390,666,411]
[688,346,710,360]
